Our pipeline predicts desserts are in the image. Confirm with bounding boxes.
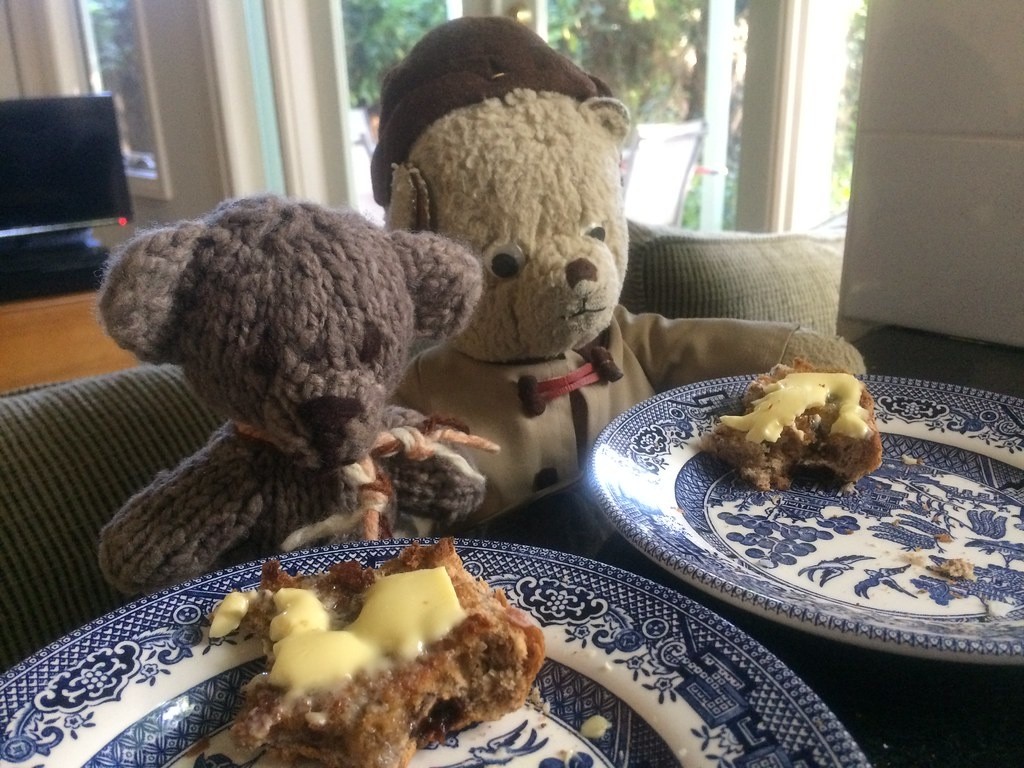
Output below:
[705,365,883,486]
[235,539,543,768]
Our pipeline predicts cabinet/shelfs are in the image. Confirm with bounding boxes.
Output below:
[0,294,140,393]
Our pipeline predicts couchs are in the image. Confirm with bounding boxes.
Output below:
[0,217,844,674]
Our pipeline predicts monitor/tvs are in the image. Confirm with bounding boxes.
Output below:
[0,93,133,238]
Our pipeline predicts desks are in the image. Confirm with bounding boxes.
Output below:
[475,326,1024,768]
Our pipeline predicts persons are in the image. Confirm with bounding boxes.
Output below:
[349,104,388,225]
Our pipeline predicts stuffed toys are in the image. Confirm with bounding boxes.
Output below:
[369,14,868,530]
[95,195,488,607]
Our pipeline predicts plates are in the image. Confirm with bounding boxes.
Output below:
[0,537,872,768]
[587,370,1024,664]
